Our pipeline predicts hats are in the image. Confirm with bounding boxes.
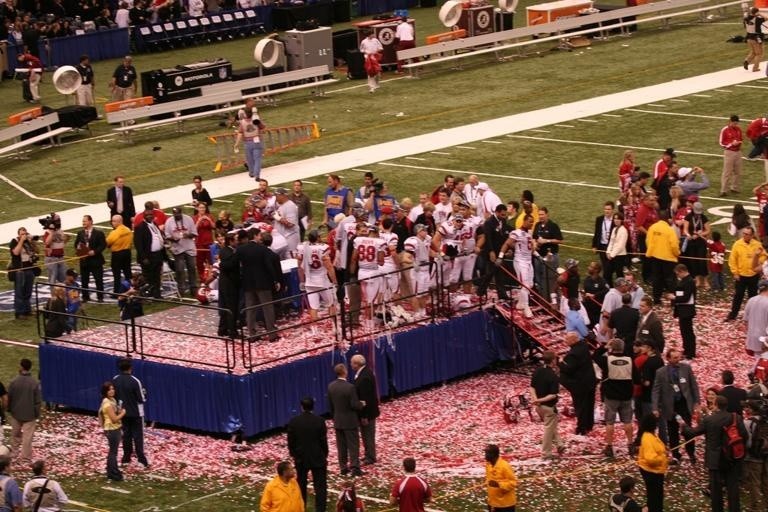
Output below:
[678,167,693,178]
[381,206,393,215]
[273,187,288,195]
[414,224,429,233]
[759,335,768,345]
[455,215,463,222]
[473,182,489,191]
[309,230,323,243]
[457,201,471,209]
[566,258,579,270]
[67,268,81,277]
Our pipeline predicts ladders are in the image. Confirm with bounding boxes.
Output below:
[207,123,320,173]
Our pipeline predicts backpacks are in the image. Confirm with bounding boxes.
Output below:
[98,401,115,427]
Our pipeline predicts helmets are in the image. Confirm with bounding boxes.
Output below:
[693,202,703,214]
[196,286,213,303]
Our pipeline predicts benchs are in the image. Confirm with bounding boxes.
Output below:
[397,0,753,80]
[200,64,339,116]
[0,112,72,159]
[106,90,246,146]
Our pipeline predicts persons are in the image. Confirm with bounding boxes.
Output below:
[0,0,768,512]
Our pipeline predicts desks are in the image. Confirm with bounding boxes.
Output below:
[273,2,332,31]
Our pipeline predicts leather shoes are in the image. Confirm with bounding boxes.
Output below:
[341,456,375,475]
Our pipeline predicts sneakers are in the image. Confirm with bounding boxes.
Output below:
[501,296,534,319]
[311,333,324,343]
[413,314,432,324]
[601,447,636,457]
[17,313,37,320]
[219,331,280,342]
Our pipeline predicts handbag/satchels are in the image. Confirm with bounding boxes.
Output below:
[722,425,747,463]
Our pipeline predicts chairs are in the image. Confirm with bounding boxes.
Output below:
[139,9,267,54]
[30,16,118,36]
